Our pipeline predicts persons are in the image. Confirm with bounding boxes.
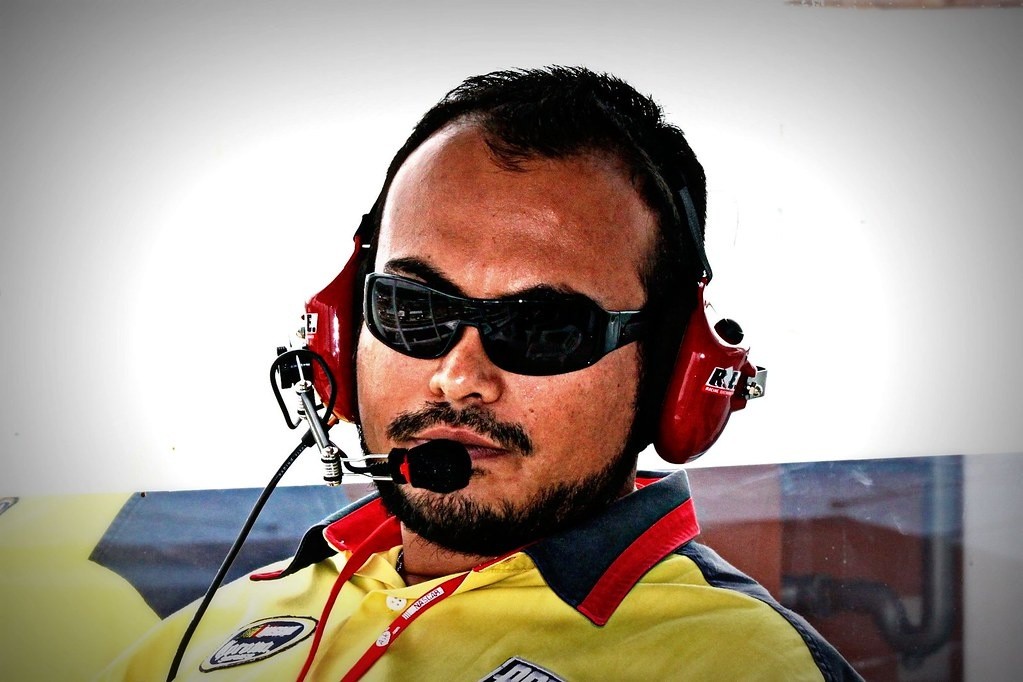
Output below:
[90,64,863,682]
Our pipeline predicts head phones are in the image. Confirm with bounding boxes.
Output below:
[270,143,766,468]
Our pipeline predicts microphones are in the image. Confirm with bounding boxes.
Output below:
[277,343,474,494]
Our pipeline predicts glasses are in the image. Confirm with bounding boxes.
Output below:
[361,272,643,377]
